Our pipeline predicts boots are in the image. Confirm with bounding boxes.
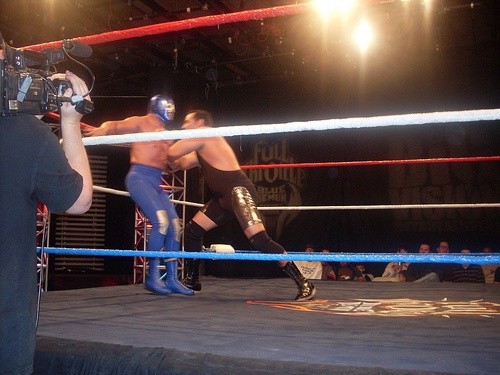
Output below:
[164,260,194,295]
[146,258,171,294]
[181,258,201,291]
[281,259,317,301]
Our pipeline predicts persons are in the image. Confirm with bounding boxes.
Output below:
[0,46,93,375]
[166,108,317,301]
[81,93,196,297]
[289,241,500,283]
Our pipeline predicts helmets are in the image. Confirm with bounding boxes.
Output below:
[148,93,176,122]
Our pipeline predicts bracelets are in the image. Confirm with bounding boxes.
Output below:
[168,166,172,171]
